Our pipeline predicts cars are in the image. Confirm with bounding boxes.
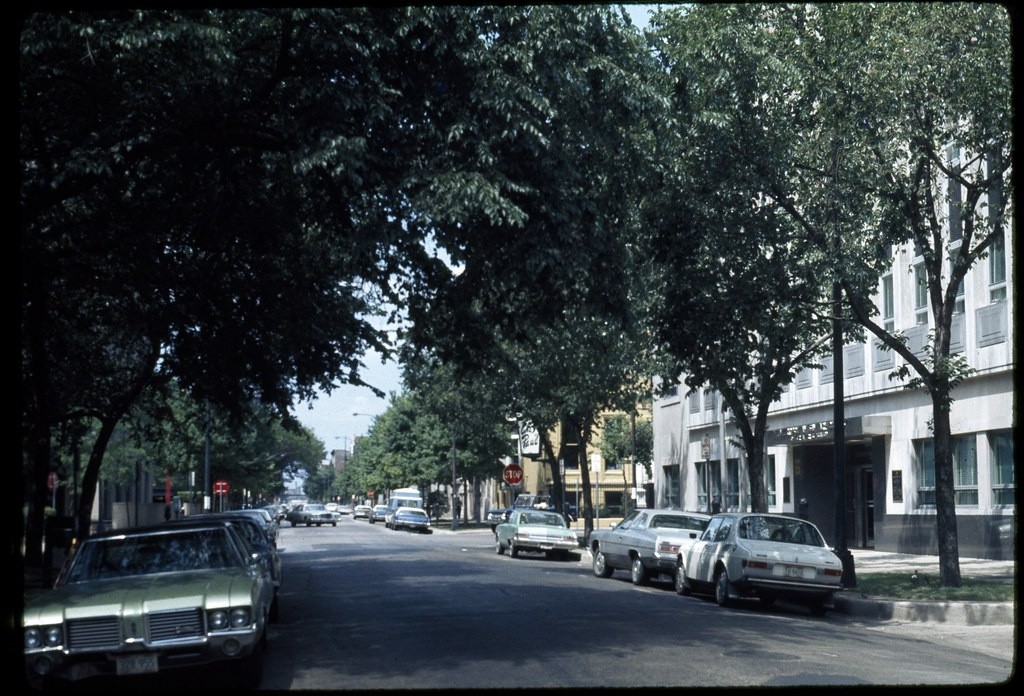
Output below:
[354,505,372,519]
[153,486,174,502]
[285,504,341,527]
[676,513,844,615]
[369,505,387,523]
[162,503,289,621]
[390,507,431,533]
[20,521,273,696]
[326,503,350,515]
[385,497,423,528]
[495,509,579,560]
[589,509,712,586]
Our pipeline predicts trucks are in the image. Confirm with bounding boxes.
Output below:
[486,494,581,534]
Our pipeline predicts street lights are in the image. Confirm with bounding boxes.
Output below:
[334,436,351,441]
[353,413,374,418]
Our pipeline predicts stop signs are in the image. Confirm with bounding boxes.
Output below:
[503,464,524,486]
